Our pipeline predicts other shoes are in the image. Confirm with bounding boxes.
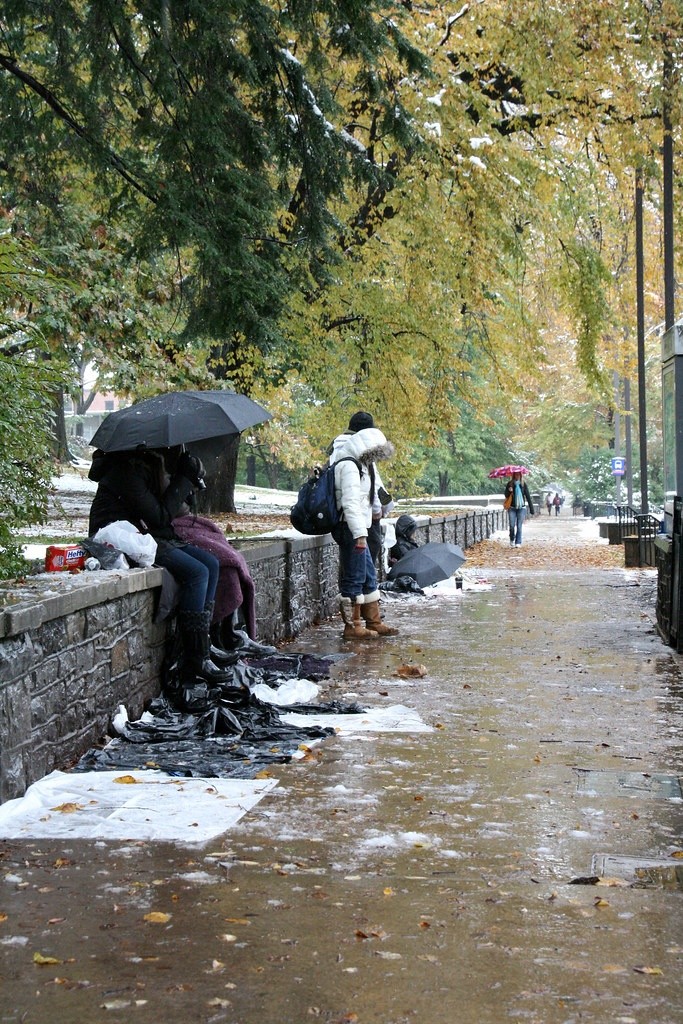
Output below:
[235,637,278,660]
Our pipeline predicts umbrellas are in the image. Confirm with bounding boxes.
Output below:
[88,391,272,506]
[487,464,529,481]
[389,542,466,589]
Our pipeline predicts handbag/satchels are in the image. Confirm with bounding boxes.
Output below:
[94,520,158,567]
[503,493,512,510]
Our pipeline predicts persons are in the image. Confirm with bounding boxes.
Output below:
[504,472,534,547]
[89,425,278,715]
[545,492,581,516]
[328,412,399,640]
[391,514,419,561]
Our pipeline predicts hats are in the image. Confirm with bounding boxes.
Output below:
[347,411,377,432]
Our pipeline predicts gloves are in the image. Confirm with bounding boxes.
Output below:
[288,456,364,535]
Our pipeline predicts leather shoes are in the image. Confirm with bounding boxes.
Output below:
[207,635,240,668]
[197,655,234,684]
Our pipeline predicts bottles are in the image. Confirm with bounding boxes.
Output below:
[84,556,101,571]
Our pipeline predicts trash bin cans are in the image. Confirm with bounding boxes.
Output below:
[531,494,540,504]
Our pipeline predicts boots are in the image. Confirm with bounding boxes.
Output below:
[360,590,399,636]
[338,596,379,640]
